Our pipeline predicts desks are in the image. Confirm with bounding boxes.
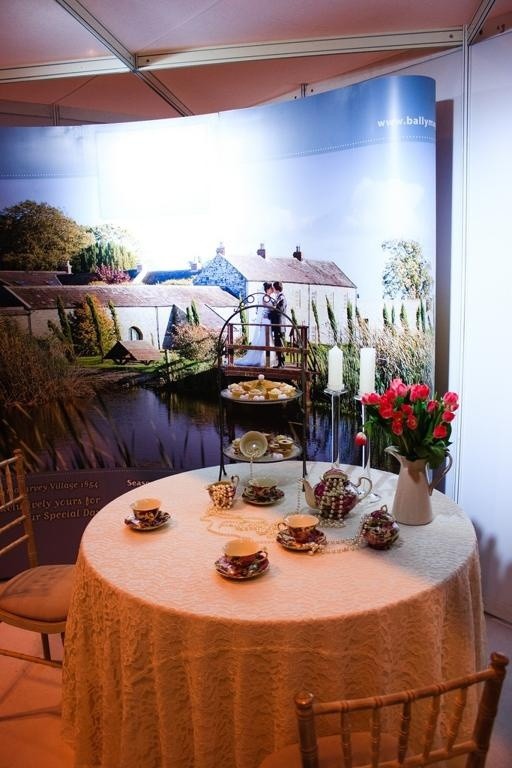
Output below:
[80,461,478,768]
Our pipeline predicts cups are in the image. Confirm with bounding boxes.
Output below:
[243,478,278,500]
[130,498,162,523]
[240,431,269,459]
[278,440,293,449]
[222,538,268,568]
[207,475,240,511]
[278,514,320,541]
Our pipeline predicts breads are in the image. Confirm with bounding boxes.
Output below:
[232,434,293,456]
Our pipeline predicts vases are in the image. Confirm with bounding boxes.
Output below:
[384,446,453,525]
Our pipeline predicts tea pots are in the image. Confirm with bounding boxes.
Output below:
[298,468,372,520]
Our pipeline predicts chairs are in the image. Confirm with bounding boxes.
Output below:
[260,650,509,768]
[0,449,75,669]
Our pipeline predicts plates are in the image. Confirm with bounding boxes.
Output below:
[213,553,270,580]
[124,510,171,531]
[276,528,326,551]
[242,488,285,506]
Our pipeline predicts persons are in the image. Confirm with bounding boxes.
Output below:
[235,282,276,367]
[265,282,287,370]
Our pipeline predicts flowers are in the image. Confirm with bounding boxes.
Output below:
[354,377,460,470]
[96,263,131,285]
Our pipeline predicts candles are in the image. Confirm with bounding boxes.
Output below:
[328,346,343,392]
[360,348,375,399]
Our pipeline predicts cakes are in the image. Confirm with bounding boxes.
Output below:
[228,380,296,400]
